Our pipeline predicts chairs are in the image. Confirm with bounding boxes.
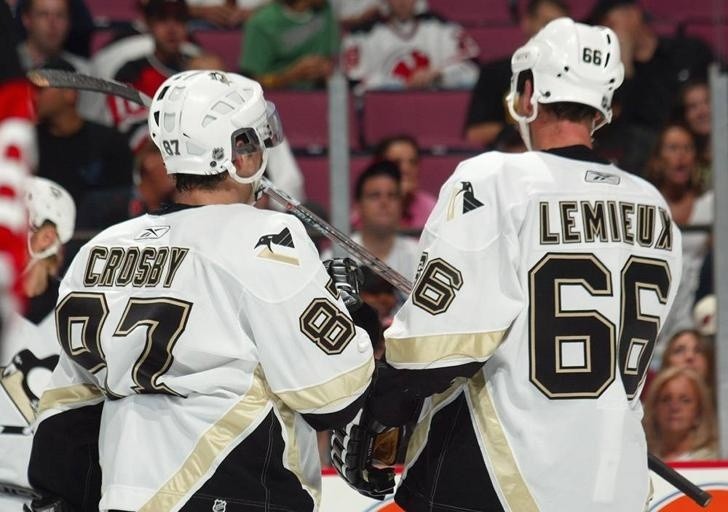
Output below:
[81,0,727,240]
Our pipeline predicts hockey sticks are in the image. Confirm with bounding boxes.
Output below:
[25,66,713,507]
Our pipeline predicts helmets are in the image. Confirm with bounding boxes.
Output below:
[149,69,283,182]
[506,17,625,128]
[27,177,75,257]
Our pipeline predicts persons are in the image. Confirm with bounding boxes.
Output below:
[11,0,96,76]
[359,0,479,108]
[678,78,714,163]
[1,172,76,506]
[645,369,718,461]
[100,1,212,218]
[694,294,717,339]
[328,16,682,512]
[344,133,436,235]
[660,328,717,388]
[24,60,133,278]
[582,2,713,164]
[26,68,374,512]
[352,262,405,356]
[237,1,340,90]
[461,2,570,150]
[321,165,423,304]
[645,127,715,228]
